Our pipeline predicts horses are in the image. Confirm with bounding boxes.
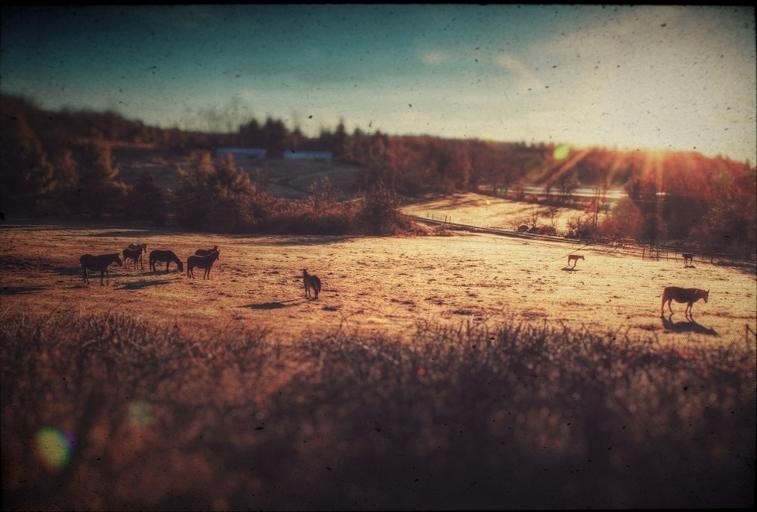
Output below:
[187,249,221,279]
[302,267,320,299]
[128,243,147,266]
[195,246,218,268]
[568,255,585,266]
[123,248,142,269]
[660,286,710,319]
[80,253,122,286]
[149,249,183,273]
[682,253,696,264]
